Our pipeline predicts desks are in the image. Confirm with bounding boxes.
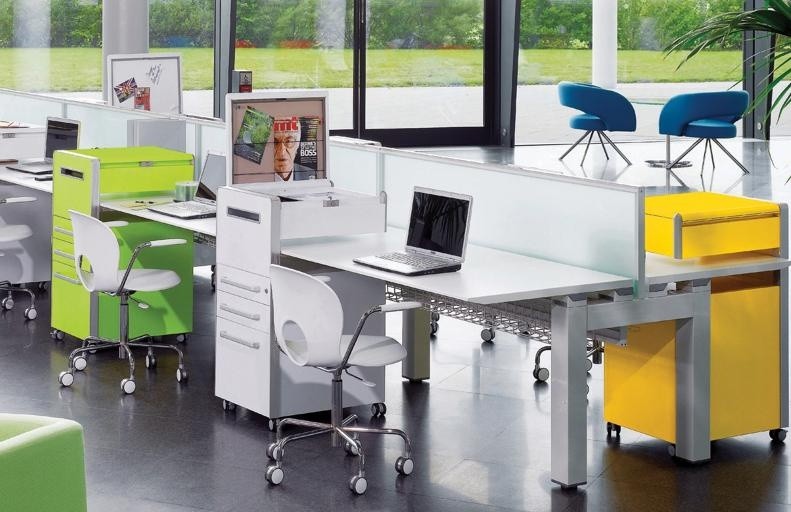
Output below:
[282,232,635,304]
[100,194,215,236]
[0,160,53,193]
[631,99,692,168]
[644,252,791,284]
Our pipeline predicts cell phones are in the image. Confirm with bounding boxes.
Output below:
[35,176,52,180]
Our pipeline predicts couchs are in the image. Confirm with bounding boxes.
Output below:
[1,413,86,511]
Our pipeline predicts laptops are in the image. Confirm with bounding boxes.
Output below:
[146,152,227,220]
[353,185,473,276]
[5,115,81,174]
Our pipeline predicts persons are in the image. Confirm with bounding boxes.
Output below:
[273,119,316,183]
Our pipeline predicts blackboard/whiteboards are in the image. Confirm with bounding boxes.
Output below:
[107,54,182,116]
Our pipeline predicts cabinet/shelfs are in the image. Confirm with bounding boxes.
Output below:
[51,145,195,353]
[603,192,788,454]
[217,182,387,430]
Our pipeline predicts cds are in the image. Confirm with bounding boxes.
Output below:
[175,181,199,201]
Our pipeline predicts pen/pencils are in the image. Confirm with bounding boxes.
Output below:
[136,201,156,204]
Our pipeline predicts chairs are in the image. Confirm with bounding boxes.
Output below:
[59,209,187,393]
[265,264,422,495]
[658,91,749,174]
[1,197,49,320]
[557,80,639,169]
